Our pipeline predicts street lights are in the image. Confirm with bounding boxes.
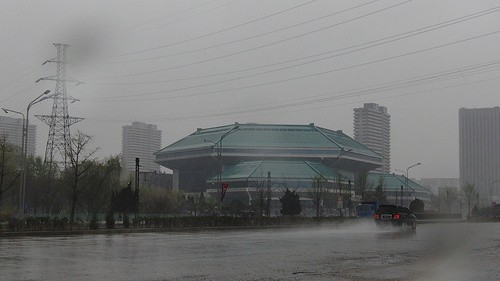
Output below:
[205,122,240,217]
[1,88,50,230]
[407,162,422,204]
[332,148,353,196]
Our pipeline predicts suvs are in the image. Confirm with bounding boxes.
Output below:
[374,205,416,231]
